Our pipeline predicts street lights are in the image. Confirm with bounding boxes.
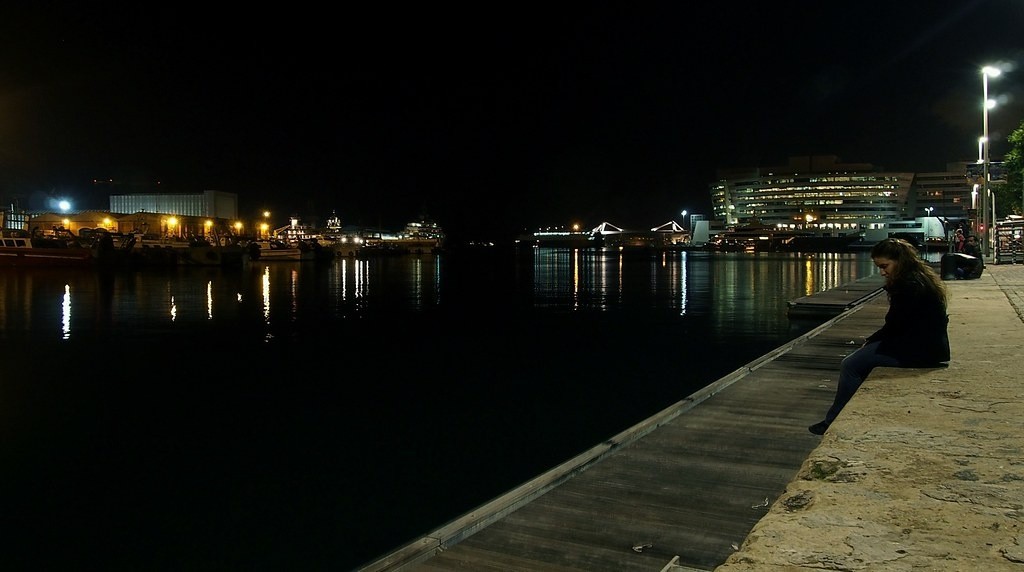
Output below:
[925,207,934,238]
[981,66,1001,258]
[977,136,990,251]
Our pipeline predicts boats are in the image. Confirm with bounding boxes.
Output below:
[0,229,101,266]
[251,247,304,262]
[186,245,250,266]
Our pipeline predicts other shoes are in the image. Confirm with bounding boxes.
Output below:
[809,420,829,435]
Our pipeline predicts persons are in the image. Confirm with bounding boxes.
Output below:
[956,229,986,269]
[809,238,950,434]
